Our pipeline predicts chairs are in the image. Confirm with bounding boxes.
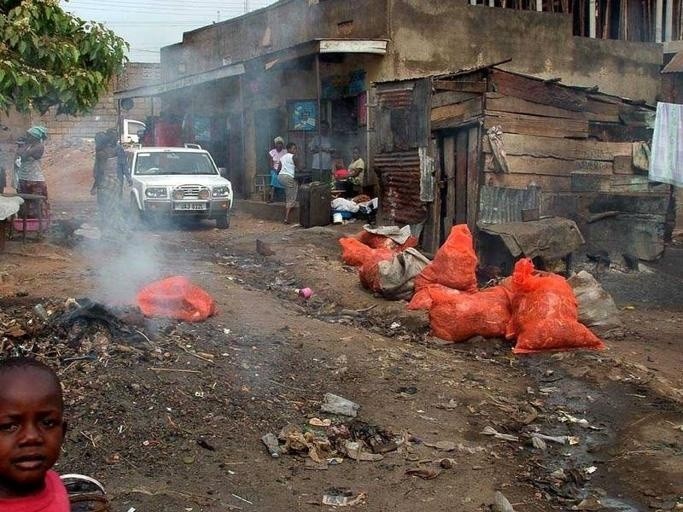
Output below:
[253,173,266,199]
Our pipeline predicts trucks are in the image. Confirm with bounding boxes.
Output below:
[82,118,148,145]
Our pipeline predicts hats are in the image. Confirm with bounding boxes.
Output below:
[16,138,26,144]
[273,136,285,148]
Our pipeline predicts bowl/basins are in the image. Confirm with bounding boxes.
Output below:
[11,218,47,232]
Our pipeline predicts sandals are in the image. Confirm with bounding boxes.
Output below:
[284,218,290,223]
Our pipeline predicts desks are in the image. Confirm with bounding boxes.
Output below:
[0,193,48,246]
[255,174,272,202]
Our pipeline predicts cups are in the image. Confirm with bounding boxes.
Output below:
[299,287,312,300]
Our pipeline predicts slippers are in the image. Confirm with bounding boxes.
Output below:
[267,200,275,203]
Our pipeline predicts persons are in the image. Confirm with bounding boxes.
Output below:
[331,147,365,198]
[0,353,72,510]
[90,128,133,239]
[266,136,286,204]
[307,119,335,181]
[276,140,304,226]
[296,112,314,131]
[13,126,52,219]
[90,131,128,196]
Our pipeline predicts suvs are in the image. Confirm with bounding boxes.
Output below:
[124,141,234,230]
